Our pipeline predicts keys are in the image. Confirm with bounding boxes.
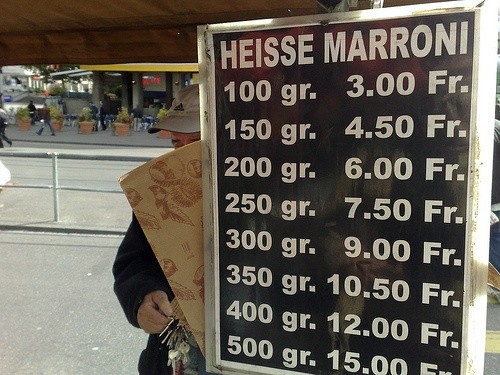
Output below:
[158,316,191,375]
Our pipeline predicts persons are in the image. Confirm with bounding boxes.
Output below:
[35,102,55,136]
[27,99,36,125]
[115,101,170,131]
[99,100,107,130]
[487,118,500,304]
[89,102,98,131]
[111,83,221,375]
[0,88,15,147]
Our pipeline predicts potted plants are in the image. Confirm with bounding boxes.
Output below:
[16,109,31,130]
[156,108,172,139]
[49,105,63,131]
[113,106,132,135]
[77,107,96,134]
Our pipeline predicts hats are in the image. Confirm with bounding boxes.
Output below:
[147,83,200,134]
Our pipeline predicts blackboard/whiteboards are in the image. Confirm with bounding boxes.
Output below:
[198,0,498,375]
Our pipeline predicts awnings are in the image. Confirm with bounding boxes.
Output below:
[80,64,199,72]
